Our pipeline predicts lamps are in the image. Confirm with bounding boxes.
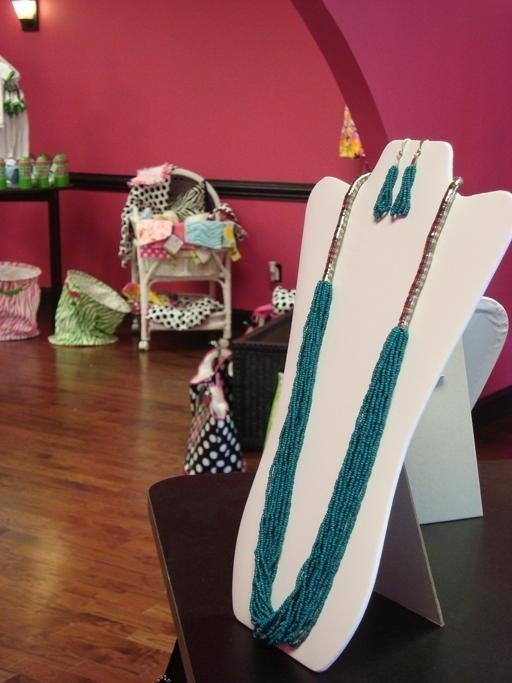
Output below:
[12,0,39,31]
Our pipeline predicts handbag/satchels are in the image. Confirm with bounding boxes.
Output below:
[185,349,246,477]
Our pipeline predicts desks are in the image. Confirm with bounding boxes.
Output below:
[146,458,512,683]
[1,183,75,332]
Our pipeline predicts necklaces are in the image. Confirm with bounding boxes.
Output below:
[250,138,463,649]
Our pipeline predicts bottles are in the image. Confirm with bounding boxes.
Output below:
[53,153,71,188]
[18,155,33,190]
[0,155,9,190]
[35,154,52,190]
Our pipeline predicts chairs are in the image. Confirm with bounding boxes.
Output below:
[127,167,235,351]
